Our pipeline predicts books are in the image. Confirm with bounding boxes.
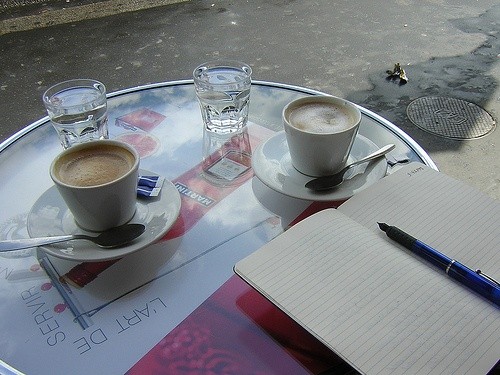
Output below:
[232,161,500,375]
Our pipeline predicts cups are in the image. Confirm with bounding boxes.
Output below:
[43,78,110,150]
[48,140,140,231]
[281,95,362,176]
[192,59,252,134]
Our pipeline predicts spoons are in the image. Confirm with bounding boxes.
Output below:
[0,224,146,252]
[304,144,395,190]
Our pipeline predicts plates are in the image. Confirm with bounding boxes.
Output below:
[27,167,182,263]
[250,129,388,202]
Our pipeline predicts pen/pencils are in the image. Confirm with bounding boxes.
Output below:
[375,220,500,306]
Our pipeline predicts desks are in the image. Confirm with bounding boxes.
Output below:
[0,80,441,375]
